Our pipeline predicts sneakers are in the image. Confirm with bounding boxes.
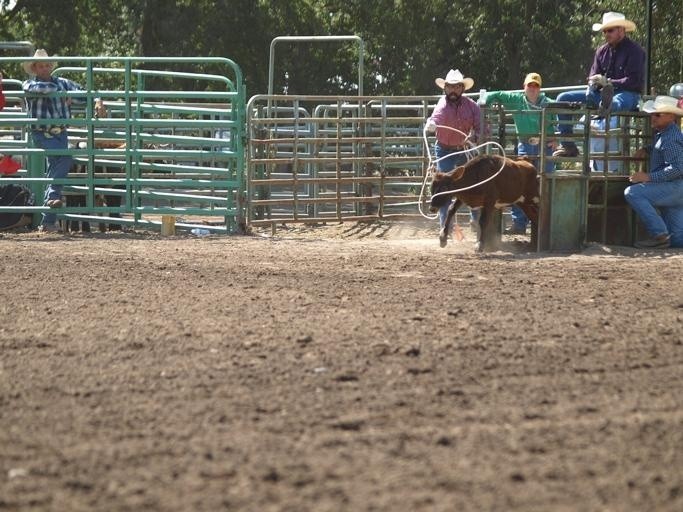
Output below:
[441,228,451,238]
[634,235,671,248]
[597,84,614,119]
[48,199,62,207]
[552,148,579,157]
[505,222,526,234]
[42,224,61,232]
[470,223,478,232]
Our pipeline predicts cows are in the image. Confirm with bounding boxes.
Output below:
[58,139,170,233]
[428,153,539,254]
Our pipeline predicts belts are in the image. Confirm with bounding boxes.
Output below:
[520,137,553,144]
[439,142,469,150]
[50,127,66,134]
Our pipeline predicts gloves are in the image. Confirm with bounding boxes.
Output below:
[589,74,609,85]
[471,143,478,153]
[427,120,436,132]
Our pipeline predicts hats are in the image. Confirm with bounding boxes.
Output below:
[435,69,474,90]
[525,73,542,86]
[643,96,683,116]
[592,12,635,33]
[23,49,57,77]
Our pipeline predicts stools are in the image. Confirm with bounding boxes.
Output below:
[658,206,683,247]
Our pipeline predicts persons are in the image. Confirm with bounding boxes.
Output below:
[20,49,106,234]
[550,10,648,155]
[477,74,562,237]
[573,115,623,172]
[623,96,683,252]
[424,67,482,242]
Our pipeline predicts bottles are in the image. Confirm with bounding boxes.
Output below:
[190,229,210,238]
